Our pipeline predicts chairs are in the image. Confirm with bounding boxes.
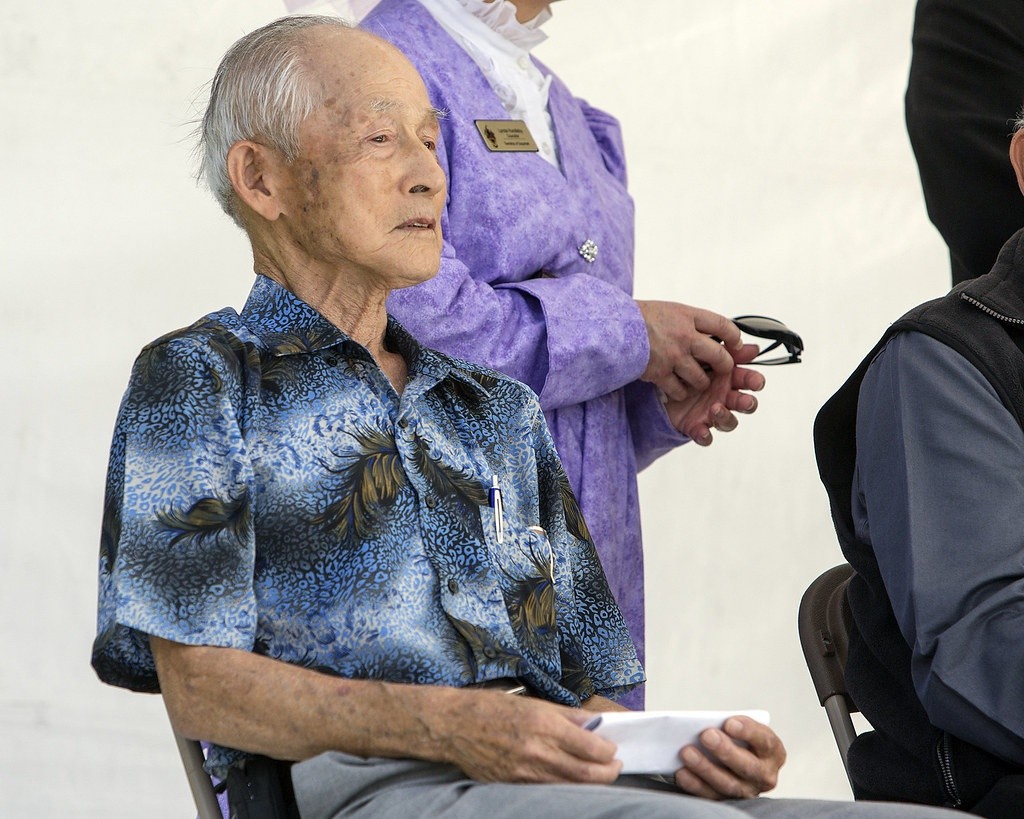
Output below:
[799,565,933,801]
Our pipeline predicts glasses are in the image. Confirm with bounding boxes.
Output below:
[701,316,804,371]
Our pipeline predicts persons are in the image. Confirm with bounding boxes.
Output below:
[352,2,767,714]
[134,15,987,819]
[809,0,1024,819]
